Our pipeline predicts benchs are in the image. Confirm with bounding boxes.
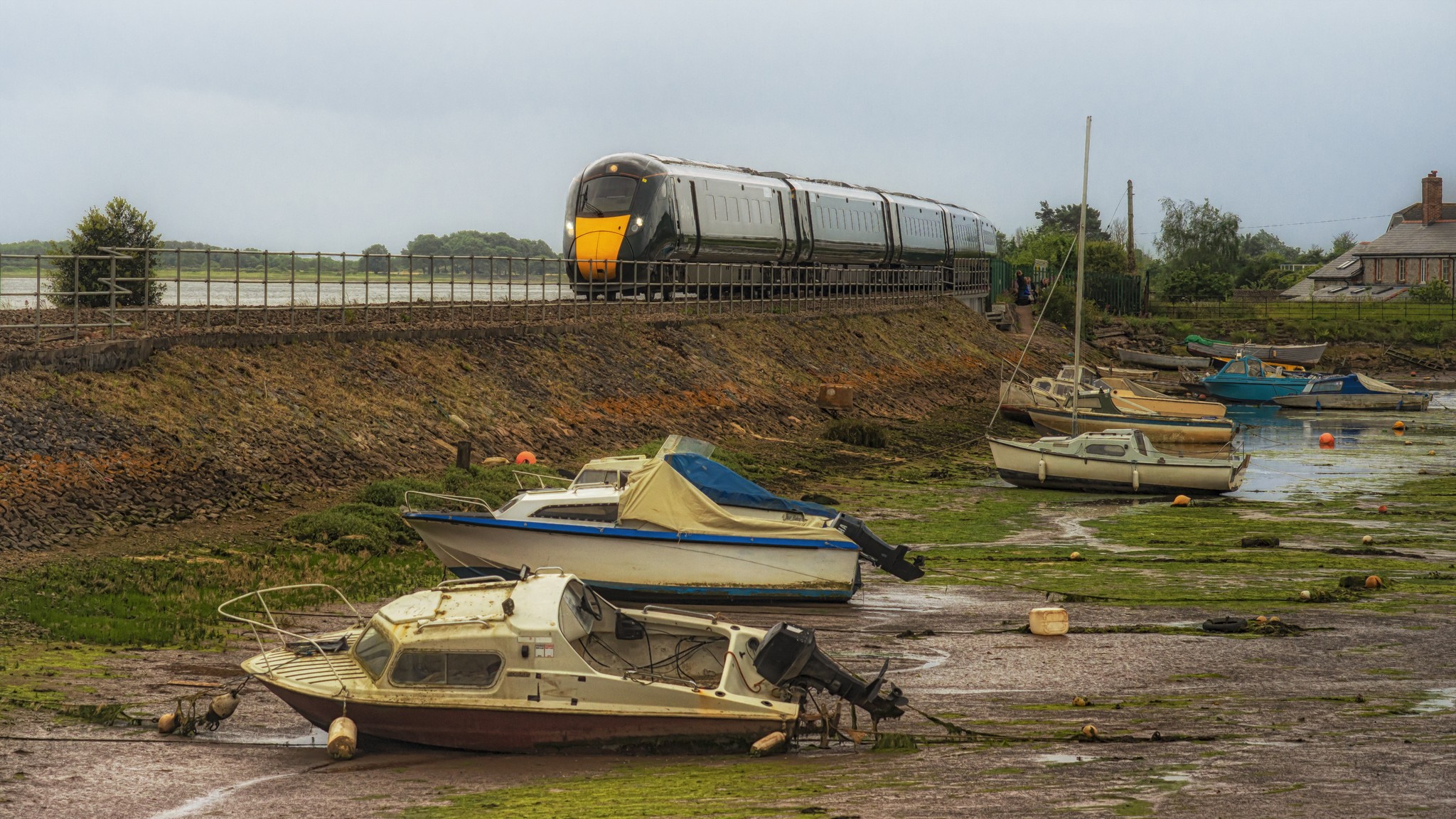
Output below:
[983,310,1014,331]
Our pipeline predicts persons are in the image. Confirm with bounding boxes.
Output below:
[1038,278,1050,297]
[1016,270,1027,298]
[1023,277,1038,304]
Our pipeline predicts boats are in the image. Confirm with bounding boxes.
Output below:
[1184,331,1329,369]
[397,434,931,603]
[1268,372,1434,411]
[1113,345,1214,370]
[1212,356,1306,377]
[1025,387,1238,445]
[216,564,910,759]
[994,361,1236,445]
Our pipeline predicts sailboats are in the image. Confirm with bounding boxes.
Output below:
[984,114,1252,494]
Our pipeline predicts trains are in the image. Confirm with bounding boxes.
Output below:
[562,153,1001,298]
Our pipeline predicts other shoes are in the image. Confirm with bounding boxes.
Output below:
[1028,295,1034,301]
